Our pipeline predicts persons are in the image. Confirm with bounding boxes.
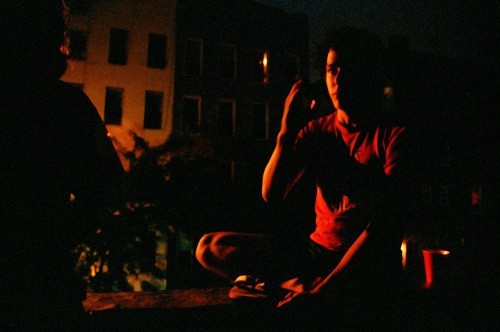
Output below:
[0,0,130,332]
[196,24,417,308]
